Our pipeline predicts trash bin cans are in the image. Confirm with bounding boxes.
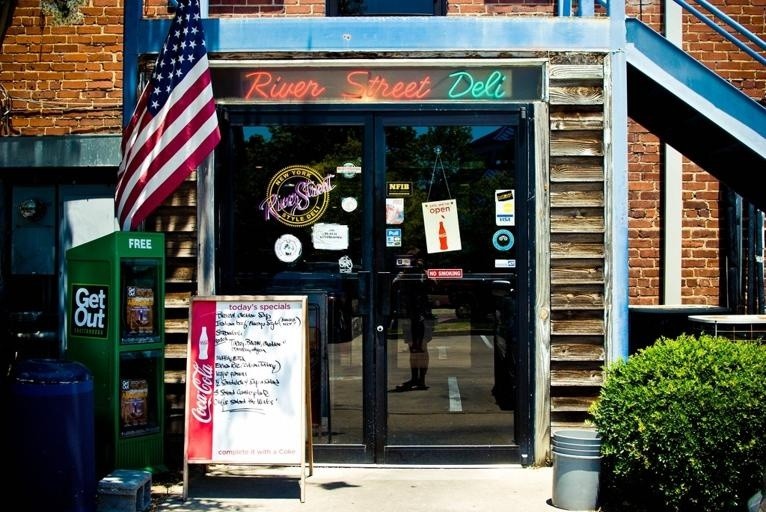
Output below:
[0,359,96,512]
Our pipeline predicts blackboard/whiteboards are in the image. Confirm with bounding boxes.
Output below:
[184,295,307,466]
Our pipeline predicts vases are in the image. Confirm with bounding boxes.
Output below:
[552,429,601,510]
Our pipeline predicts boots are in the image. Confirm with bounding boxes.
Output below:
[402,367,427,390]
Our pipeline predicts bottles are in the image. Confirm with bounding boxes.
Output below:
[438,222,449,251]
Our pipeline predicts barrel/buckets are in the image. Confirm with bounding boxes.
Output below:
[550,430,603,511]
[14,359,97,512]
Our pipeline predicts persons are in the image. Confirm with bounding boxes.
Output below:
[386,248,433,391]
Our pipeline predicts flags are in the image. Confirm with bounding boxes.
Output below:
[113,0,223,232]
